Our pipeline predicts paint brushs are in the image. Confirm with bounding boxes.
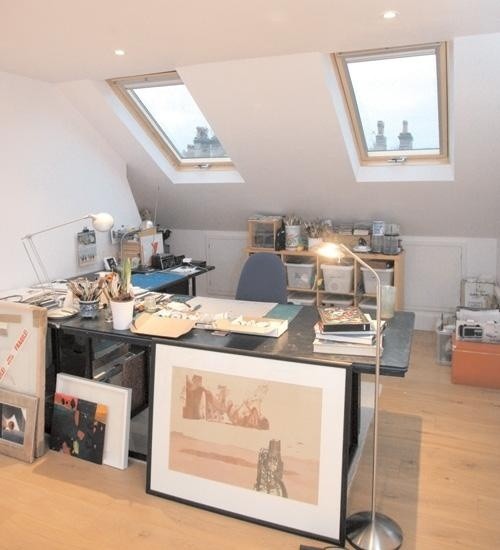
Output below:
[69,273,122,301]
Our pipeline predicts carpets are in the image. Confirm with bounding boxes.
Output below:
[128,380,383,502]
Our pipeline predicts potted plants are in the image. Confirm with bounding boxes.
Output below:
[109,259,135,330]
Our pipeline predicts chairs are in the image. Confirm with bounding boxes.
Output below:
[235,251,289,301]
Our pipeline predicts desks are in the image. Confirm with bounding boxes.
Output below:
[1,265,415,464]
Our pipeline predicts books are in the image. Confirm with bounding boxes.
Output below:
[312,305,386,356]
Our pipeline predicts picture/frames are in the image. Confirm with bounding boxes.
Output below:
[1,387,40,464]
[144,337,354,548]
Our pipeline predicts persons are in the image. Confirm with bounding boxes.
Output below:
[5,421,17,432]
[58,424,105,461]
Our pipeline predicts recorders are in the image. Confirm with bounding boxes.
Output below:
[153,253,176,270]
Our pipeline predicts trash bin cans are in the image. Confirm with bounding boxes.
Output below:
[436,319,452,364]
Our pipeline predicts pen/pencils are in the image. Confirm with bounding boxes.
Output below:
[192,305,200,312]
[282,213,323,237]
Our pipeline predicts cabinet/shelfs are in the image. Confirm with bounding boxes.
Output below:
[242,246,405,312]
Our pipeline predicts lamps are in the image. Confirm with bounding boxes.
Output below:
[315,241,404,550]
[20,213,115,320]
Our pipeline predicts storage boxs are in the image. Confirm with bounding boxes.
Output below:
[360,261,394,294]
[436,312,457,365]
[320,261,354,293]
[451,331,500,390]
[284,259,314,288]
[461,279,496,308]
[287,292,314,306]
[321,294,354,307]
[359,296,376,309]
